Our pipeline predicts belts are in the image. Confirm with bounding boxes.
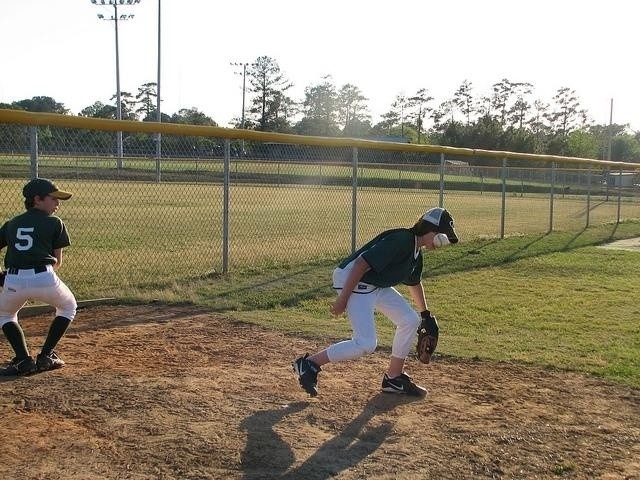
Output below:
[8,266,47,275]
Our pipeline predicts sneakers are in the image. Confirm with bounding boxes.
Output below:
[36,352,65,370]
[0,356,37,375]
[382,372,427,396]
[291,352,322,397]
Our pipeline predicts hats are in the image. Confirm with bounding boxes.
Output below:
[421,208,458,243]
[24,177,72,200]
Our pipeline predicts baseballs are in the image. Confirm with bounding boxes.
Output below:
[434,233,449,248]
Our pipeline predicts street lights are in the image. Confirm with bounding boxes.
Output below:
[90,0,142,169]
[229,62,259,157]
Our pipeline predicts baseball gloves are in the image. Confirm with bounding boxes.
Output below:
[416,310,439,364]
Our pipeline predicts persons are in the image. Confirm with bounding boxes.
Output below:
[0,178,78,376]
[292,207,459,397]
